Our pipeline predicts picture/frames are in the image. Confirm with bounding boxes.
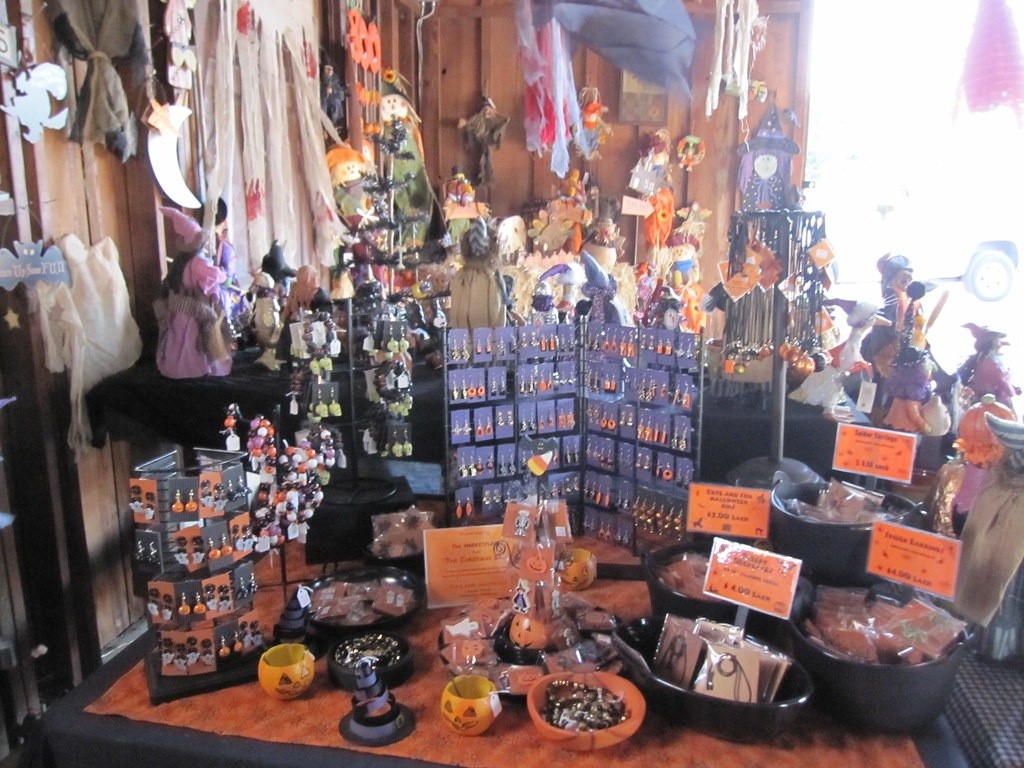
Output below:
[618,71,668,127]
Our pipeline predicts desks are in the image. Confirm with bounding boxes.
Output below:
[17,491,969,768]
[86,365,836,485]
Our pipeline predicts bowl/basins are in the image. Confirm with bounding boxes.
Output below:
[529,674,645,749]
[771,472,922,587]
[293,567,422,636]
[787,592,975,732]
[613,618,814,745]
[642,547,799,633]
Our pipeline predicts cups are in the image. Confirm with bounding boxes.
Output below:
[258,644,315,699]
[442,675,497,736]
